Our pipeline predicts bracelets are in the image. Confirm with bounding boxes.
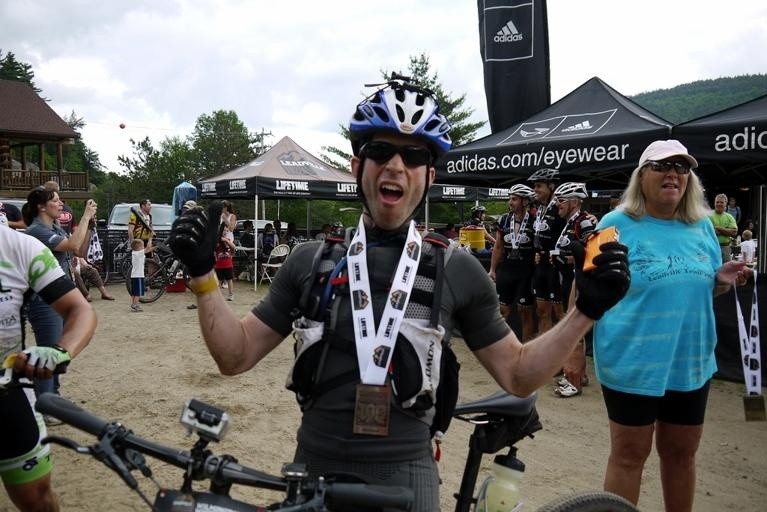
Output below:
[191,272,219,297]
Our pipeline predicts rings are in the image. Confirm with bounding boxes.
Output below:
[741,280,746,285]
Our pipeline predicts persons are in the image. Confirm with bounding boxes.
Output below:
[489,169,623,397]
[238,221,343,282]
[710,193,756,267]
[0,213,98,512]
[128,200,152,313]
[213,199,236,301]
[443,206,496,258]
[0,181,115,302]
[165,81,631,511]
[1,139,25,179]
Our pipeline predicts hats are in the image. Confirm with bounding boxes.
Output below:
[637,140,698,172]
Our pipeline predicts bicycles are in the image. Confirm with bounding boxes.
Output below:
[33,393,642,512]
[120,236,175,278]
[125,246,196,303]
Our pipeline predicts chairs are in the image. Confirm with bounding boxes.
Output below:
[259,244,290,286]
[238,240,263,277]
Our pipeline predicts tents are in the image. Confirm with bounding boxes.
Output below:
[672,95,767,274]
[201,136,358,291]
[424,76,673,231]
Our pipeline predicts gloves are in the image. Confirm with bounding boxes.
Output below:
[568,238,630,321]
[16,345,72,378]
[170,200,221,278]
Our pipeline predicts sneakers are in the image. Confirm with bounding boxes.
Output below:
[554,375,589,397]
[102,294,115,301]
[132,305,143,312]
[228,292,233,302]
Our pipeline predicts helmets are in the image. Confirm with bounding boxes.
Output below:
[347,79,451,163]
[509,168,590,203]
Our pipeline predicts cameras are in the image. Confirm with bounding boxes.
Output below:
[89,199,98,207]
[584,227,619,279]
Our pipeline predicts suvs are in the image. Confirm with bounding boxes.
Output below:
[0,197,77,230]
[234,219,288,231]
[94,199,182,265]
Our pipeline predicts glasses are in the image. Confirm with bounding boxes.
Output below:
[650,161,690,175]
[357,140,432,168]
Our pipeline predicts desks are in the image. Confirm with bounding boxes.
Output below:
[235,247,260,251]
